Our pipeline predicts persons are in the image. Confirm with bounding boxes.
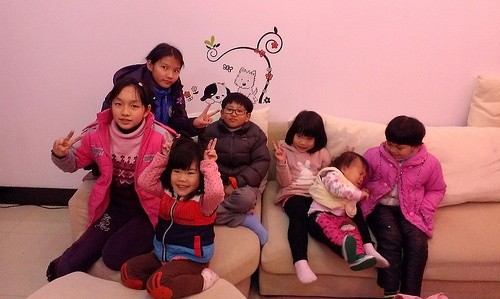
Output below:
[68,43,220,242]
[308,151,377,271]
[198,92,271,246]
[272,110,390,283]
[121,134,225,299]
[359,115,447,299]
[46,78,179,283]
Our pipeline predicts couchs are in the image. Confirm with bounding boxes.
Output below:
[29,109,500,299]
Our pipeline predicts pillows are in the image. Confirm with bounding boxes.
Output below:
[467,75,500,128]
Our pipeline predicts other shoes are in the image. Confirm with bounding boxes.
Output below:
[343,235,356,263]
[350,255,376,271]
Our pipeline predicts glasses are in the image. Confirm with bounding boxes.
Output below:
[224,107,248,115]
